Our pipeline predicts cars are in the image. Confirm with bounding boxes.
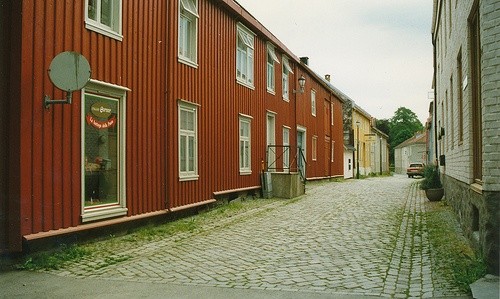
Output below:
[407,162,426,179]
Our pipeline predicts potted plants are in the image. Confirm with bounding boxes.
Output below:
[417,162,445,201]
